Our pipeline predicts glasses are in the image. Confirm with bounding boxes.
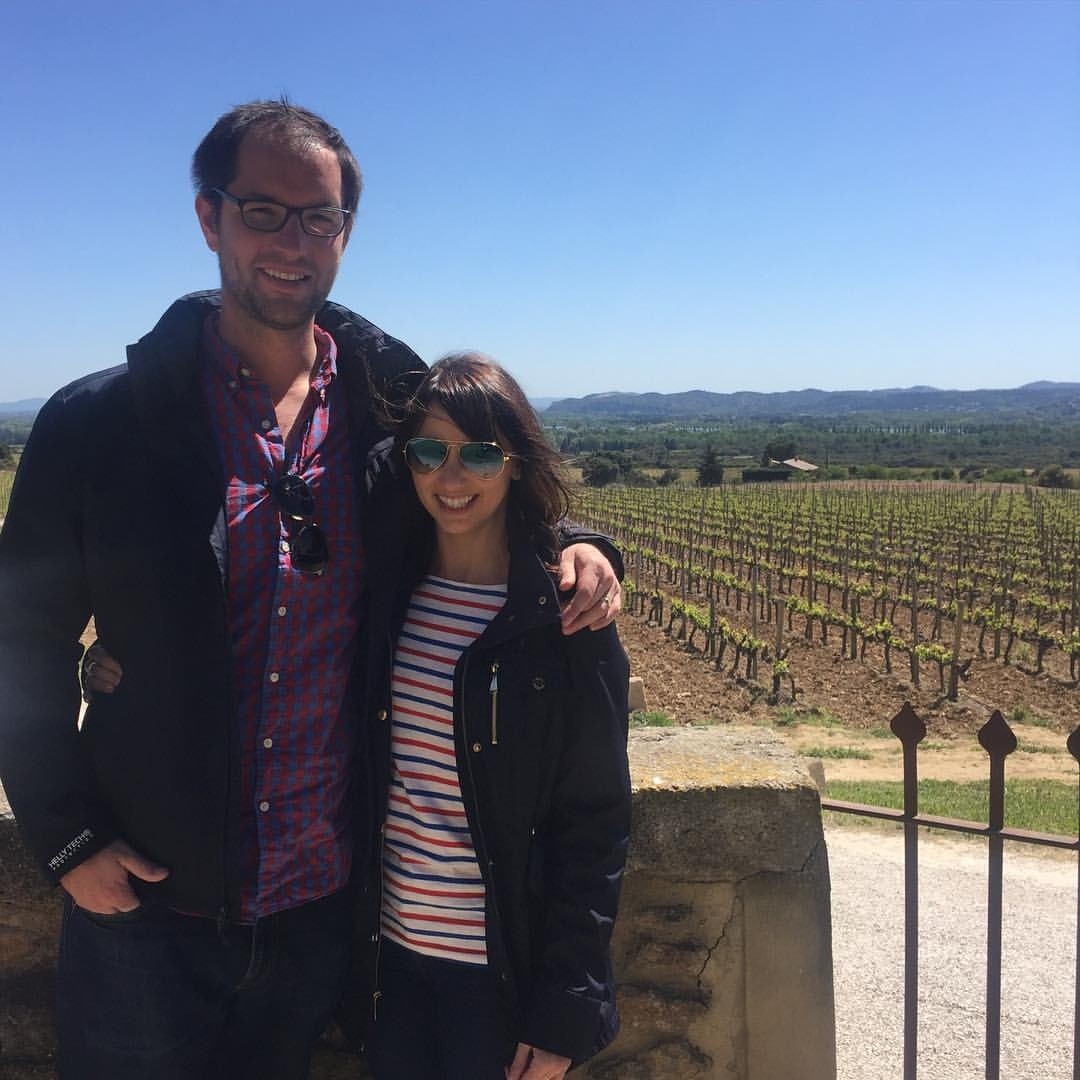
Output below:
[210,185,353,238]
[269,465,329,572]
[402,437,528,481]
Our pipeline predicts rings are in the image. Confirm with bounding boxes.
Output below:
[602,597,611,610]
[87,661,96,672]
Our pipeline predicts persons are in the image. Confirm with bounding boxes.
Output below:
[0,99,427,1080]
[81,351,633,1080]
